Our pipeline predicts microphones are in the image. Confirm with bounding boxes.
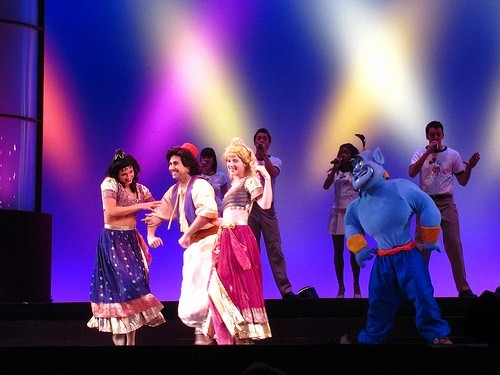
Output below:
[258,144,262,149]
[434,143,438,150]
[331,157,343,164]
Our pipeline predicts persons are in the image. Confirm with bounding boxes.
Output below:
[247,128,297,307]
[201,137,272,345]
[408,121,480,299]
[146,142,218,346]
[199,147,228,218]
[323,141,366,298]
[86,147,163,346]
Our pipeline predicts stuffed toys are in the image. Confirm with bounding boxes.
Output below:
[340,147,452,347]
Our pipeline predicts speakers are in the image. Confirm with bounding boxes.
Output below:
[0,208,53,304]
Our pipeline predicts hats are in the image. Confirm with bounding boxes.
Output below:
[182,142,198,158]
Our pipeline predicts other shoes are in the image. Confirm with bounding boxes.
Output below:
[335,287,345,298]
[458,290,477,299]
[353,286,362,298]
